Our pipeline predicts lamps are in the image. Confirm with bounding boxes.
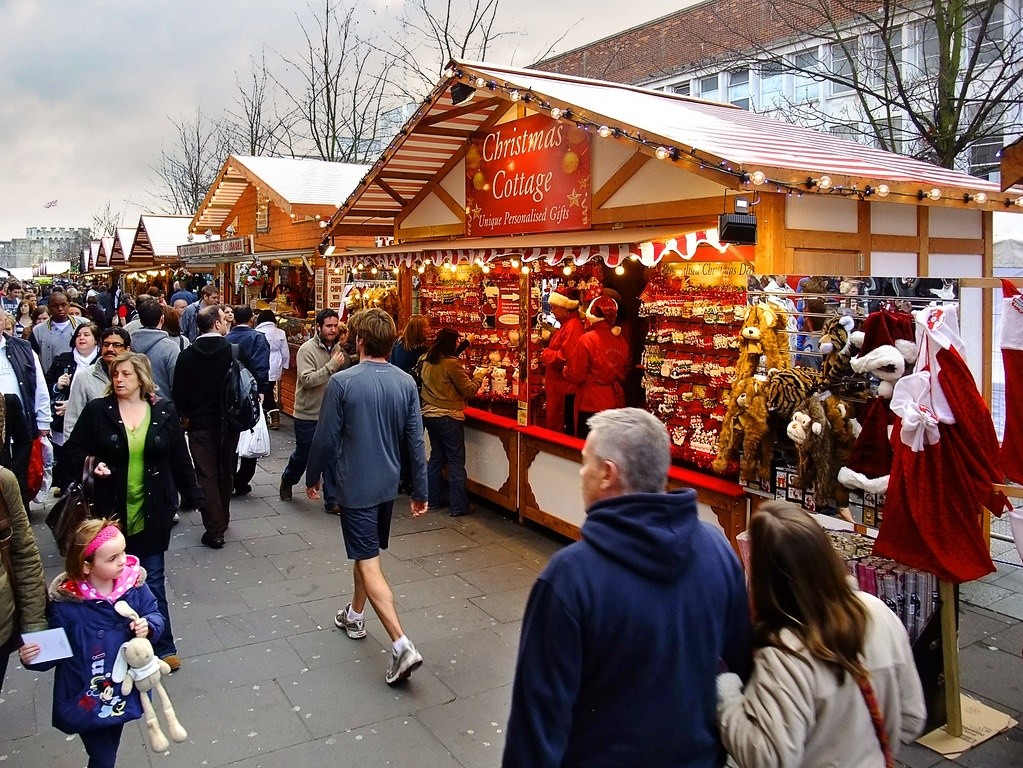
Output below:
[319,237,335,257]
[204,229,213,242]
[718,187,758,247]
[451,82,476,106]
[226,225,235,238]
[187,233,197,244]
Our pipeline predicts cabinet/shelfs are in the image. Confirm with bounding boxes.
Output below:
[746,293,964,741]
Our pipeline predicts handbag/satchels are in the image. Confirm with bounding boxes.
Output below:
[235,394,272,459]
[28,434,53,504]
[45,482,91,557]
[50,368,68,433]
[409,359,423,394]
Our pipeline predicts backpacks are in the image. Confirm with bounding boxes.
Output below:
[222,344,260,432]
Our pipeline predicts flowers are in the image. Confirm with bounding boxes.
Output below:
[238,259,270,286]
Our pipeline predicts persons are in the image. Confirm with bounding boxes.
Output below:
[0,278,493,768]
[715,498,928,768]
[563,296,629,439]
[747,274,849,371]
[537,286,583,439]
[501,409,761,768]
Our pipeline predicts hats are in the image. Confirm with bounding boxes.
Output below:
[548,287,583,309]
[850,311,918,381]
[837,381,893,494]
[586,296,621,336]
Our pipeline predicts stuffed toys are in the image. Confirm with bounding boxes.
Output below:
[112,601,188,753]
[712,307,865,524]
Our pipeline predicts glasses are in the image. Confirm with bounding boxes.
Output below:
[103,342,124,348]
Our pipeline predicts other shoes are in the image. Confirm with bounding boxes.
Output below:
[280,482,292,500]
[202,532,224,548]
[325,503,340,516]
[163,655,180,669]
[428,502,448,509]
[448,504,478,516]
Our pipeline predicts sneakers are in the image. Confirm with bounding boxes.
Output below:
[335,602,367,640]
[385,641,423,686]
